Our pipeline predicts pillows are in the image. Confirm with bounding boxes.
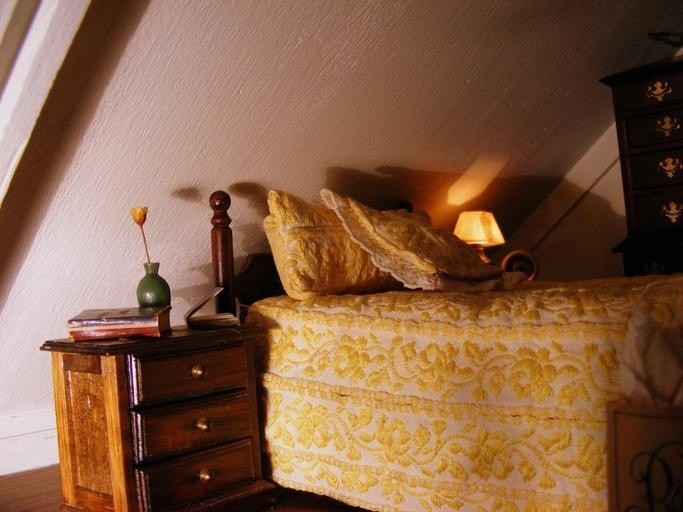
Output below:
[264,186,529,301]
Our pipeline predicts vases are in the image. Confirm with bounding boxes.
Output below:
[137,261,171,309]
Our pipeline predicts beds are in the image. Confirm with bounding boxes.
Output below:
[209,188,683,512]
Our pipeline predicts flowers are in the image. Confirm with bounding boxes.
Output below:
[128,204,156,262]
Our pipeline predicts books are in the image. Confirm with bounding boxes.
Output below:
[68,304,172,340]
[184,285,240,328]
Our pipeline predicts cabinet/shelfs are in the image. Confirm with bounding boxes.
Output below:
[597,55,683,275]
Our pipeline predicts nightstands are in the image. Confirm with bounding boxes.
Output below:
[38,326,274,511]
